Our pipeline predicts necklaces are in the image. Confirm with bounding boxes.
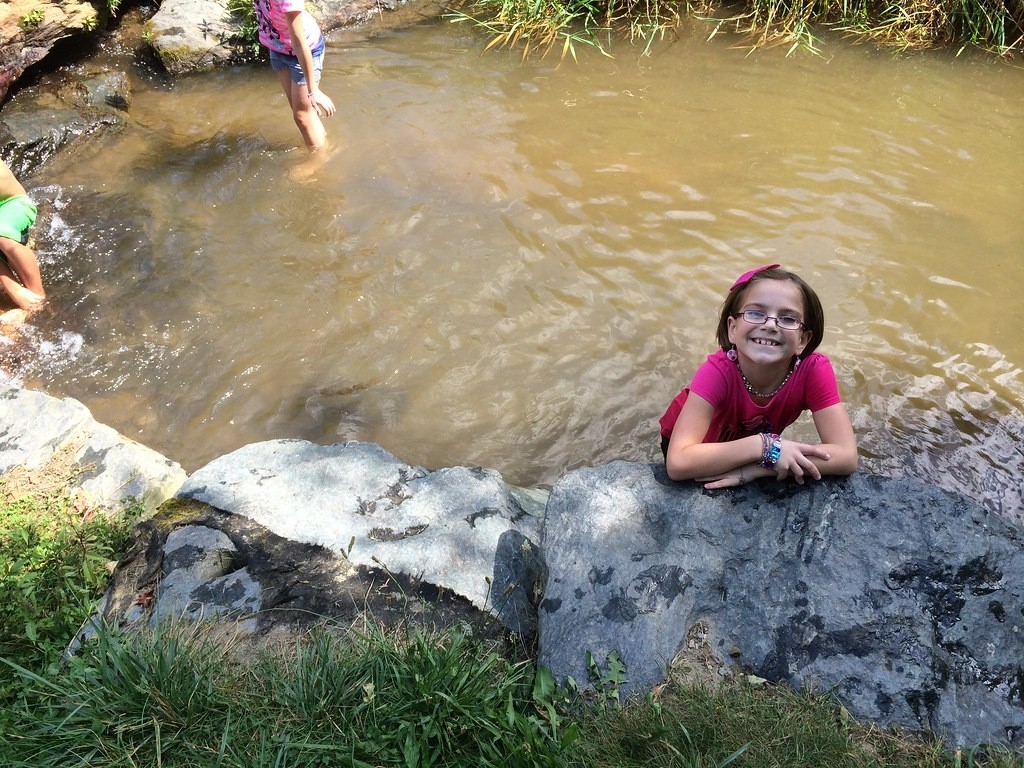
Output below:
[738,361,792,397]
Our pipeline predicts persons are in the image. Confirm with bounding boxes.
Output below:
[0,158,44,309]
[255,0,336,146]
[657,263,858,488]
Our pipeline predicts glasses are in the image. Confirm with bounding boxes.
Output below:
[731,311,809,330]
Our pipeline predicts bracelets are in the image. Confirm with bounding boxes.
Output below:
[757,433,782,470]
[308,87,317,96]
